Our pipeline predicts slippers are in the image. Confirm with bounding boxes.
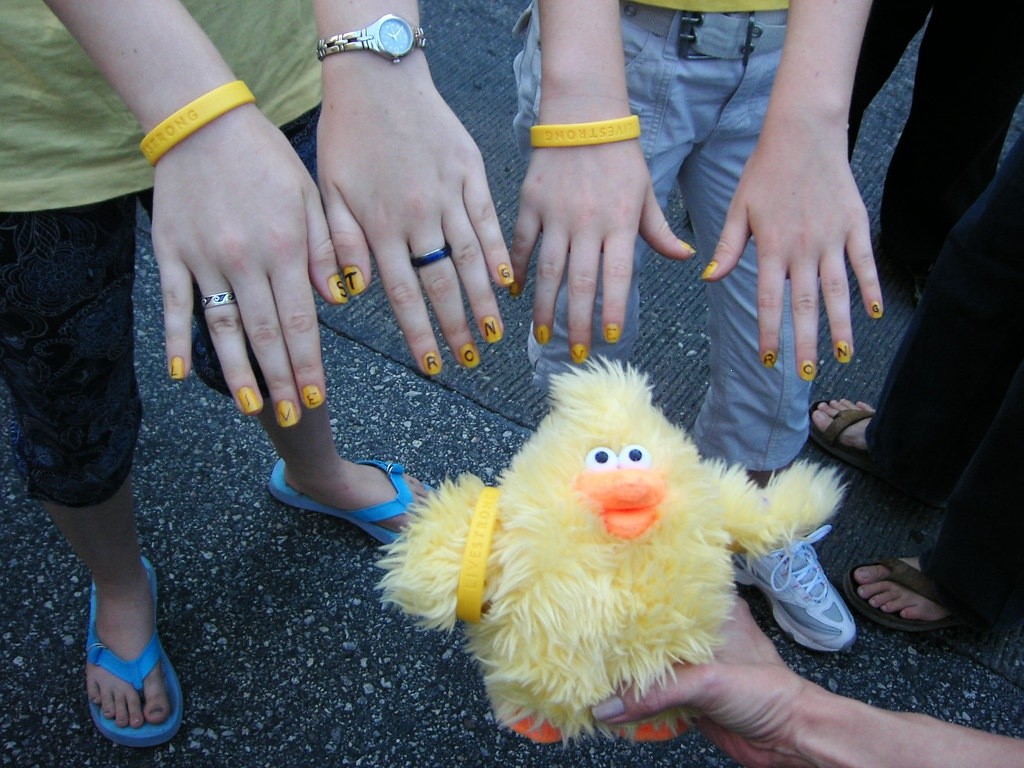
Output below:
[810,392,904,475]
[846,545,1021,632]
[82,556,183,745]
[269,451,434,546]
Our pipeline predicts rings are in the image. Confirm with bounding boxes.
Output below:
[201,291,238,308]
[411,244,452,269]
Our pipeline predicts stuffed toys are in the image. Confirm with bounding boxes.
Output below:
[373,352,849,740]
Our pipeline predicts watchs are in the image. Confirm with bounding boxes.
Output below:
[314,13,426,63]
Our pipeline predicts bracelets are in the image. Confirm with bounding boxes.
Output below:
[455,485,499,623]
[138,81,254,165]
[531,114,639,147]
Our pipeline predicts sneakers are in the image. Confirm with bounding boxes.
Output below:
[735,523,857,653]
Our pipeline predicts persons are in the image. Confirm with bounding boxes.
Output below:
[511,0,883,652]
[1,0,515,748]
[593,595,1023,768]
[807,0,1024,632]
